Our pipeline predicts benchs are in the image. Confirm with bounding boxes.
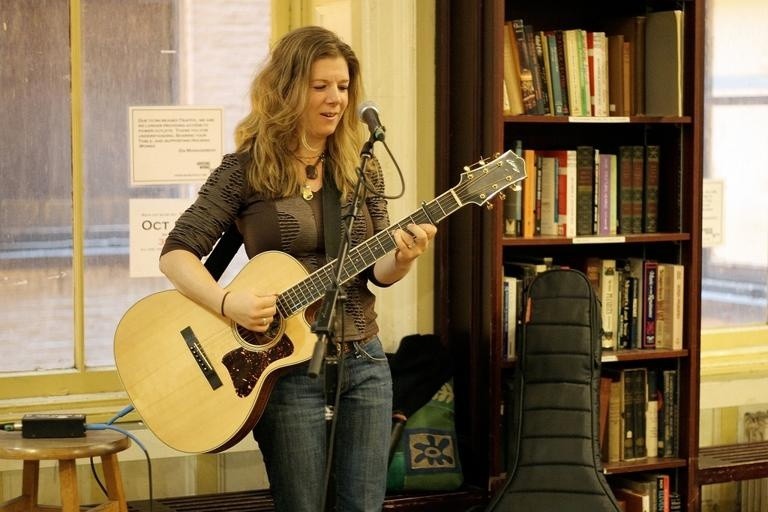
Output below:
[697,441,767,510]
[80,478,479,510]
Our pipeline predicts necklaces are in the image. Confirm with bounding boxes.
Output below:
[288,148,328,179]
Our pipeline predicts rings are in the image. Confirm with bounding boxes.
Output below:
[262,317,268,326]
[406,241,417,250]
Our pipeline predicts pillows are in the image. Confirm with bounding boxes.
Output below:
[384,372,463,489]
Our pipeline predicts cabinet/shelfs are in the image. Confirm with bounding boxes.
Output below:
[433,1,706,512]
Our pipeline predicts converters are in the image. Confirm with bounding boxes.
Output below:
[21,414,86,438]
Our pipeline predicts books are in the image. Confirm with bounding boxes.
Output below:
[503,256,685,358]
[502,9,684,117]
[502,139,660,237]
[596,367,689,512]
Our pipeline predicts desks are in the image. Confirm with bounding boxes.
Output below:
[0,421,131,510]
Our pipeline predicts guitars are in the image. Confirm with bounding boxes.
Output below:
[113,148,529,453]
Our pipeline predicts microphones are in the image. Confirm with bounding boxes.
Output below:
[358,100,386,141]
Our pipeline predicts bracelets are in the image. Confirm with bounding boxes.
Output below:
[219,290,233,321]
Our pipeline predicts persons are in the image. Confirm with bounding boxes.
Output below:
[161,26,440,511]
